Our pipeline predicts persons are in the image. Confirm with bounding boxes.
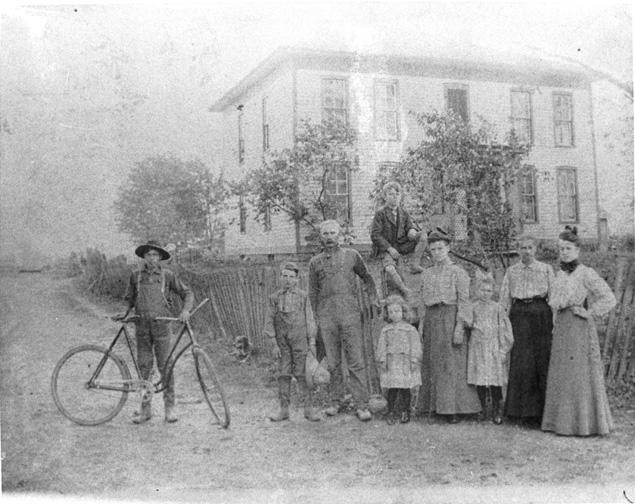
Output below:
[371,182,429,297]
[263,262,316,422]
[499,236,554,425]
[113,239,194,422]
[542,225,617,436]
[309,219,382,421]
[466,275,514,423]
[418,228,483,422]
[375,294,423,426]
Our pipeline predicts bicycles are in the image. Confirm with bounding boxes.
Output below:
[49,298,232,429]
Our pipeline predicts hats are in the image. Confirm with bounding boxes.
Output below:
[134,239,172,261]
[283,262,298,272]
[303,348,330,392]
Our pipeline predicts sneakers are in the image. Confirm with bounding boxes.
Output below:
[132,409,178,423]
[386,411,409,424]
[325,402,341,415]
[301,407,321,422]
[354,408,372,419]
[268,408,290,421]
